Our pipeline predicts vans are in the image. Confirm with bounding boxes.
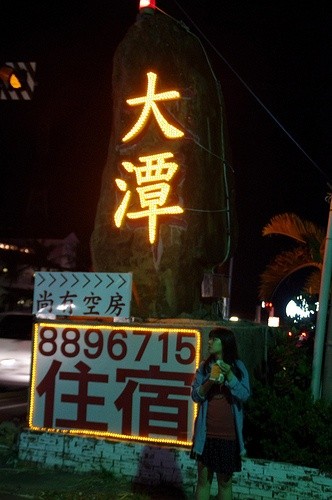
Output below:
[0,313,36,387]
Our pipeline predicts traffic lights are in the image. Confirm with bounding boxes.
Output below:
[0,61,34,102]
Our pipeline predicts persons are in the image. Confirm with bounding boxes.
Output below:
[189,327,251,500]
